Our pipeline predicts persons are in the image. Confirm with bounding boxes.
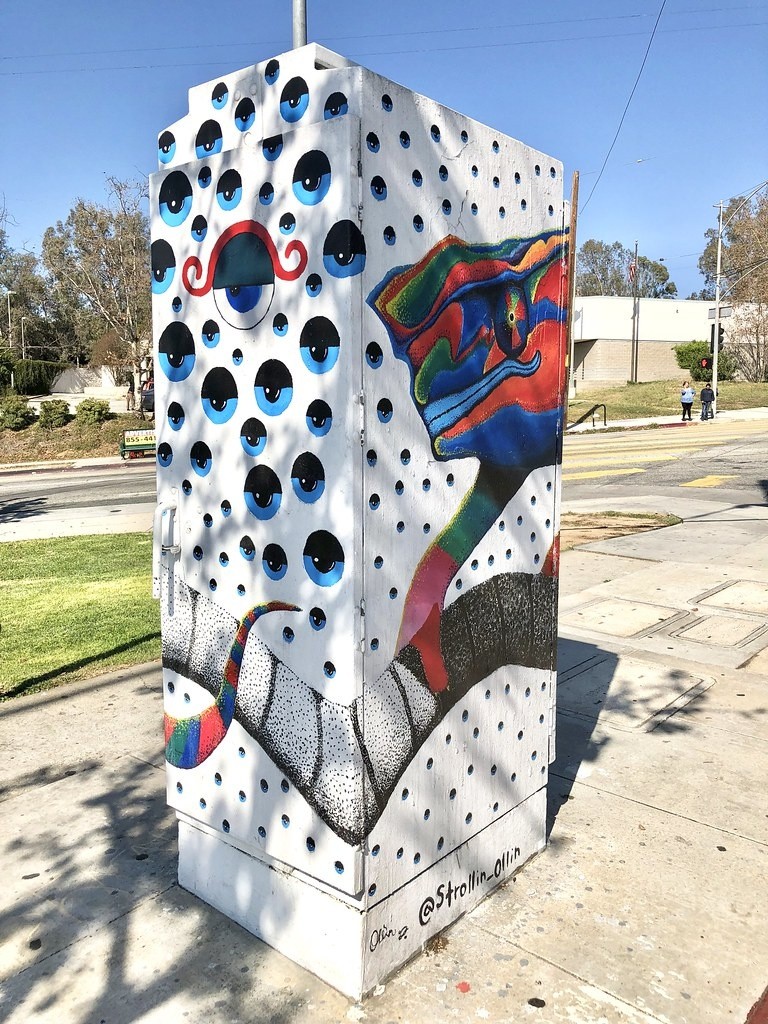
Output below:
[681,381,696,421]
[126,381,137,411]
[700,383,715,421]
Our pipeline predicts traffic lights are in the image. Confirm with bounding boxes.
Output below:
[700,357,713,368]
[710,322,725,354]
